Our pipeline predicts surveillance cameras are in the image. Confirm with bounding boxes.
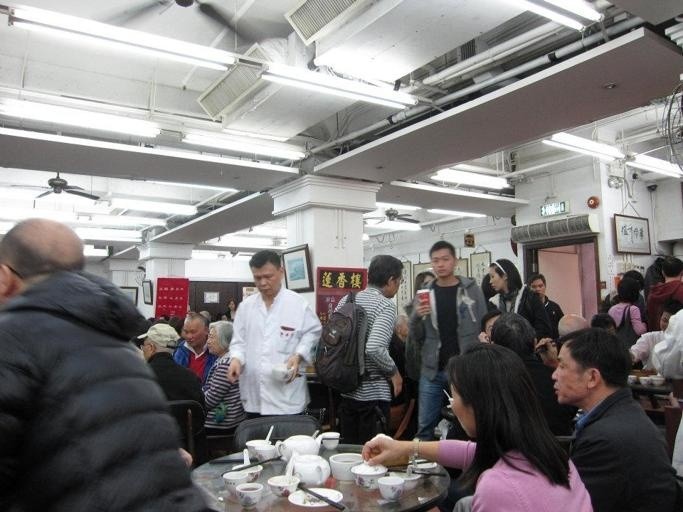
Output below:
[647,183,657,192]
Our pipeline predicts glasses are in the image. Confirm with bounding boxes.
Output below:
[1,260,26,285]
[140,345,154,354]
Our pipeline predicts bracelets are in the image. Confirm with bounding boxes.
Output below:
[412,437,420,463]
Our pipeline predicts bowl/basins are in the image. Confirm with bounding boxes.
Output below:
[389,469,422,495]
[244,439,271,459]
[270,364,294,382]
[230,463,263,482]
[650,375,665,387]
[266,474,300,499]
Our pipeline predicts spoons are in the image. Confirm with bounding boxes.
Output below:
[243,448,251,470]
[282,455,295,483]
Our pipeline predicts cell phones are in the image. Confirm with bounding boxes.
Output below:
[416,289,430,305]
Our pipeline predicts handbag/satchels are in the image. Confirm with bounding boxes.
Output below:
[618,304,645,369]
[389,397,416,440]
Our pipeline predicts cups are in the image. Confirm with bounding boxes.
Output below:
[637,376,651,387]
[328,452,364,485]
[377,476,403,503]
[351,459,388,493]
[221,470,248,498]
[415,289,431,316]
[234,482,263,510]
[321,432,340,450]
[253,445,275,465]
[626,375,637,385]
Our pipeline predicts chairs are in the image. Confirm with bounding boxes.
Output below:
[233,416,321,452]
[168,400,205,466]
[663,394,681,454]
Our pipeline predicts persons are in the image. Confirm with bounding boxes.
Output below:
[528,273,564,342]
[491,313,578,437]
[486,259,552,340]
[221,299,239,323]
[174,315,218,389]
[389,316,418,428]
[608,279,648,370]
[319,254,402,446]
[478,310,502,343]
[189,322,249,461]
[653,309,683,480]
[0,218,209,512]
[553,328,681,512]
[601,270,645,313]
[229,250,322,419]
[147,310,213,335]
[481,272,498,300]
[408,241,488,441]
[644,256,667,283]
[396,272,436,442]
[533,314,588,367]
[136,323,207,471]
[361,342,593,512]
[630,302,682,370]
[591,312,616,334]
[647,256,683,330]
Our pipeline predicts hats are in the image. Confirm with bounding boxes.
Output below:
[136,323,180,349]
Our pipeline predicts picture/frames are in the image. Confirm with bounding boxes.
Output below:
[614,214,659,254]
[282,244,314,293]
[142,281,152,305]
[470,252,490,281]
[413,258,468,298]
[396,262,412,316]
[120,286,138,306]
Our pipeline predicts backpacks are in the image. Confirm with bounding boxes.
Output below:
[316,290,370,394]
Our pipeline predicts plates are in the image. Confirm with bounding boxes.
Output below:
[407,458,437,469]
[287,487,343,509]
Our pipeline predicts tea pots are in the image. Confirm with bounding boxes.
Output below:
[291,450,330,486]
[274,433,324,462]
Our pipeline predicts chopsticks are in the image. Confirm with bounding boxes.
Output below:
[383,460,439,470]
[208,457,259,465]
[220,456,280,477]
[298,484,345,511]
[387,468,446,478]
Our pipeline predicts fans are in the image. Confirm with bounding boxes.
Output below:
[11,172,100,201]
[362,206,420,226]
